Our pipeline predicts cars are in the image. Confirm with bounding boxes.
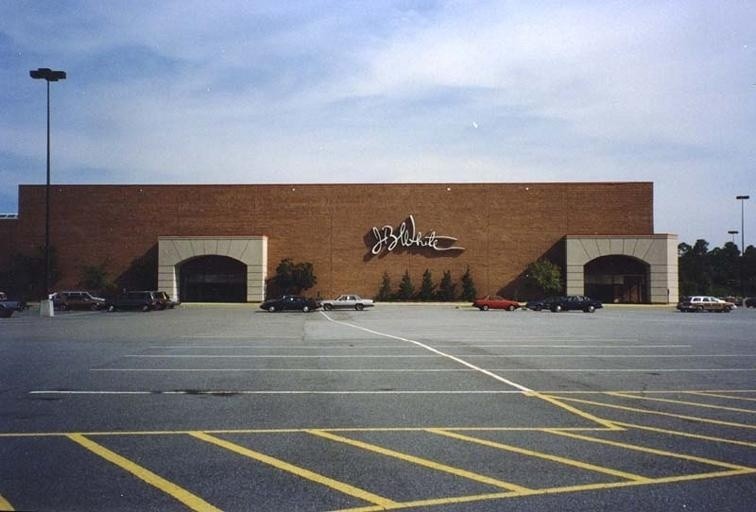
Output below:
[108,290,175,312]
[259,295,321,312]
[472,294,603,313]
[677,295,756,313]
[320,294,375,311]
[52,291,107,311]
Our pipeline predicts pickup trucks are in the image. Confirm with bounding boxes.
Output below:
[0,292,25,317]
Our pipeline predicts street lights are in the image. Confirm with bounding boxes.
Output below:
[728,230,739,243]
[29,67,67,300]
[736,195,751,256]
